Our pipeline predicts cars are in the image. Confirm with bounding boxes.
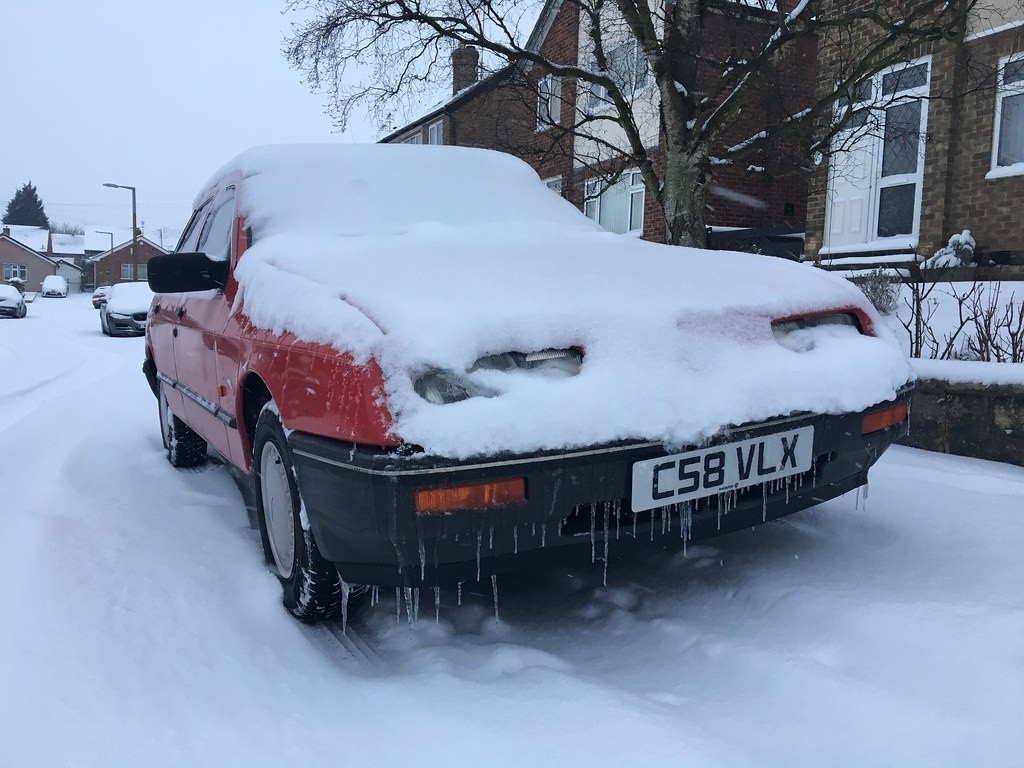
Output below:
[39,275,70,298]
[91,281,156,337]
[0,284,27,319]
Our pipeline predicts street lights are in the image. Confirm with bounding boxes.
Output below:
[95,230,115,286]
[101,183,138,282]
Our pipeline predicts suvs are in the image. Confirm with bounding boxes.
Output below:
[139,139,921,626]
